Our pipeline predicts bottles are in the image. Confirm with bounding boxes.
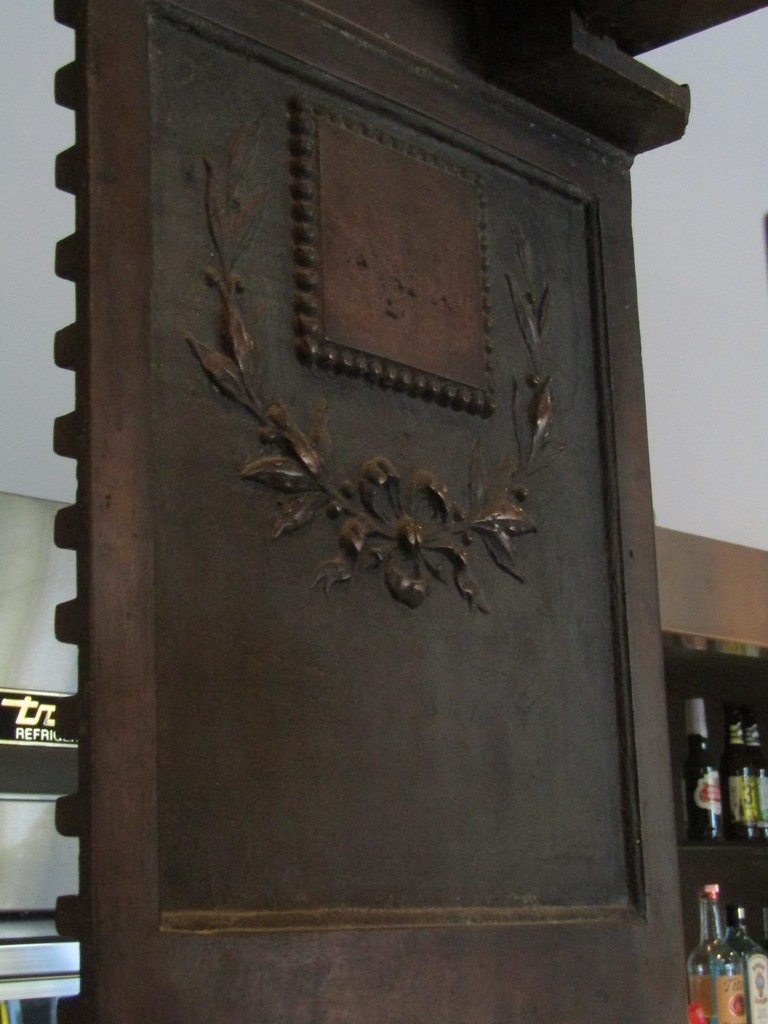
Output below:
[742,704,768,839]
[682,699,724,840]
[724,907,768,1024]
[687,883,747,1024]
[720,701,762,841]
